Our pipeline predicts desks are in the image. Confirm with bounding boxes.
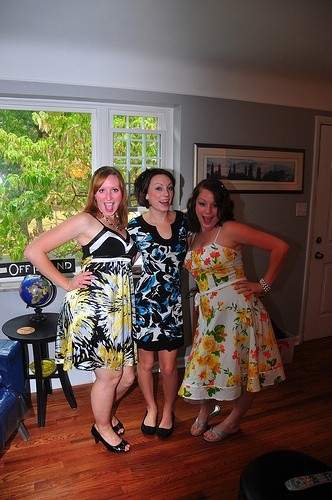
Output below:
[2,313,78,427]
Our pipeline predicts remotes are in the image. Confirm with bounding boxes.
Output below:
[285,471,332,491]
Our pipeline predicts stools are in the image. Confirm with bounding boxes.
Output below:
[238,447,332,500]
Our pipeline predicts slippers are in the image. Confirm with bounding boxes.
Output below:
[190,404,221,436]
[202,423,241,443]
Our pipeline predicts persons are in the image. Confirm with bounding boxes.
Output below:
[127,169,195,436]
[24,166,144,452]
[177,177,289,442]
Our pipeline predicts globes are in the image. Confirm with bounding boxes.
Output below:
[19,274,56,322]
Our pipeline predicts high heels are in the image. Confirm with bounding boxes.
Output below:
[91,422,130,453]
[111,416,126,437]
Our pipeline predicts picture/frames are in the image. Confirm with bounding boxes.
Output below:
[193,143,307,192]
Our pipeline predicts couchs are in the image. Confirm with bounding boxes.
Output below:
[0,339,36,447]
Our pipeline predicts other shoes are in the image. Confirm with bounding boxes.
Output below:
[141,411,158,435]
[157,412,175,438]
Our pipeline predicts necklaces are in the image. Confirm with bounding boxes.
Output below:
[103,214,123,231]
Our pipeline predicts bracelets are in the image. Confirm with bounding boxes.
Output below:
[259,278,271,293]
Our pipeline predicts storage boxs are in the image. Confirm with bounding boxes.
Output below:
[273,319,296,364]
